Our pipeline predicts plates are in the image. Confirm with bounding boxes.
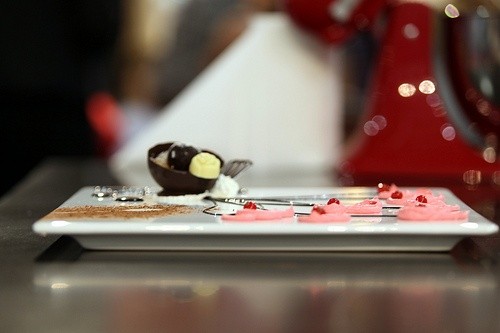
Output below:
[31,186,500,251]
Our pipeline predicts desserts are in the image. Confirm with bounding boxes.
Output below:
[148,140,224,193]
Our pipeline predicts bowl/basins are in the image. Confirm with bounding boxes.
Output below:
[148,143,225,195]
